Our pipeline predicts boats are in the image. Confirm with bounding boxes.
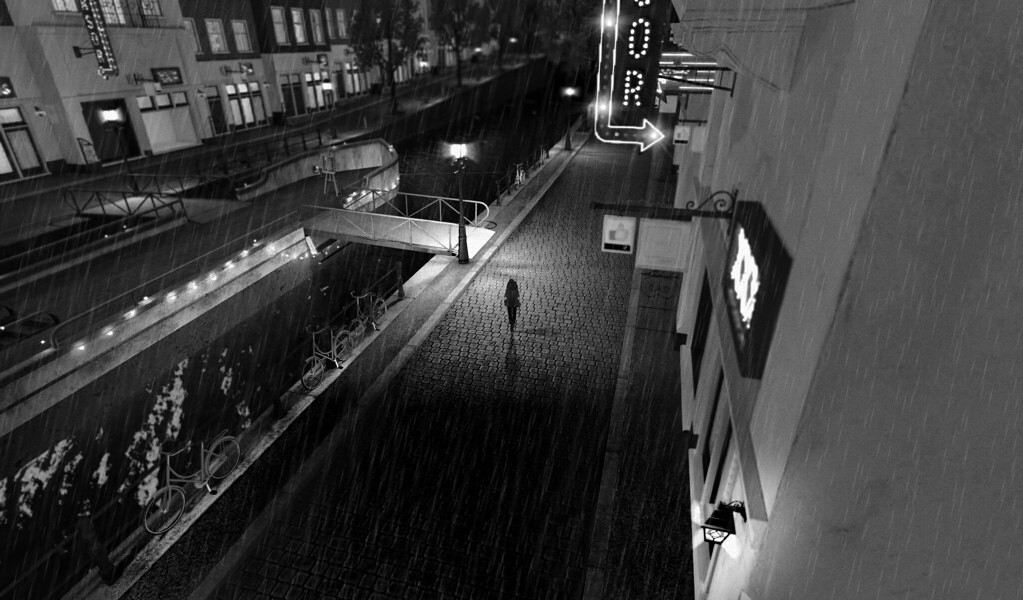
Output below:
[444,115,484,143]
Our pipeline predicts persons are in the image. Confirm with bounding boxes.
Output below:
[504,279,521,332]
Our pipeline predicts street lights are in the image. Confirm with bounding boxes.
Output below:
[565,87,574,151]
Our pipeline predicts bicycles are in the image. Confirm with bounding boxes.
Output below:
[348,290,387,348]
[140,426,241,535]
[513,162,526,190]
[301,324,354,390]
[537,144,546,167]
